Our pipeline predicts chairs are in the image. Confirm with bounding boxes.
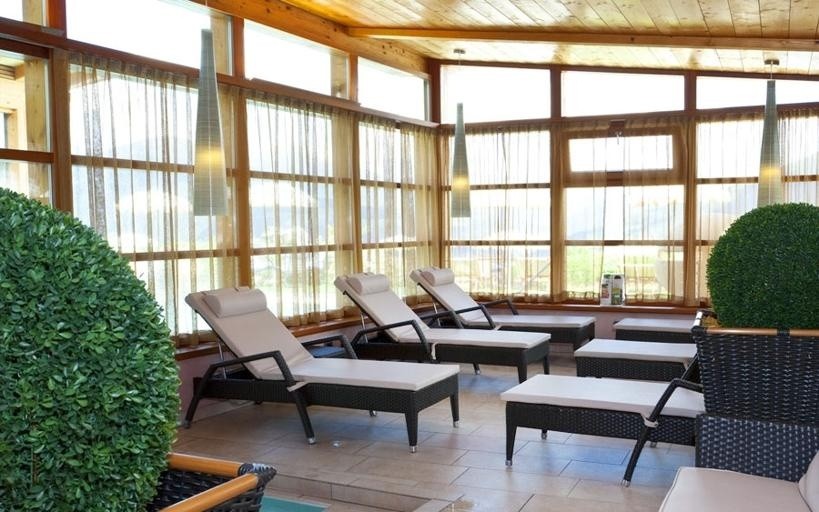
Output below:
[655,451,819,511]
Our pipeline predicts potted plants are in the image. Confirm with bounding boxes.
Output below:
[692,202,819,482]
[0,188,275,511]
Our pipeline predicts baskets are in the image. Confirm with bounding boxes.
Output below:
[691,309,819,482]
[144,453,276,510]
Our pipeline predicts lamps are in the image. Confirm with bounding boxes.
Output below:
[450,47,471,219]
[193,0,229,216]
[756,58,784,209]
